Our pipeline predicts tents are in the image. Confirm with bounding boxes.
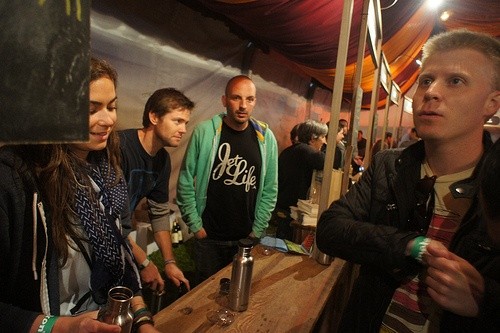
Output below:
[0,0,500,333]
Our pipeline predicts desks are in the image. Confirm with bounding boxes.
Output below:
[151,243,348,333]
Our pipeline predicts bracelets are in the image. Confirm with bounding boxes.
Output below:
[411,237,429,262]
[164,258,176,263]
[37,315,57,333]
[139,257,151,270]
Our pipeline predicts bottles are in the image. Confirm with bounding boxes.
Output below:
[317,251,332,265]
[171,221,179,247]
[227,247,254,311]
[176,221,183,240]
[97,287,135,333]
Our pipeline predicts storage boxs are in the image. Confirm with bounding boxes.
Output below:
[127,211,174,255]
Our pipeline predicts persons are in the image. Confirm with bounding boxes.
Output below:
[276,119,329,243]
[176,75,278,289]
[106,88,194,289]
[317,31,500,333]
[0,53,159,333]
[319,119,419,191]
